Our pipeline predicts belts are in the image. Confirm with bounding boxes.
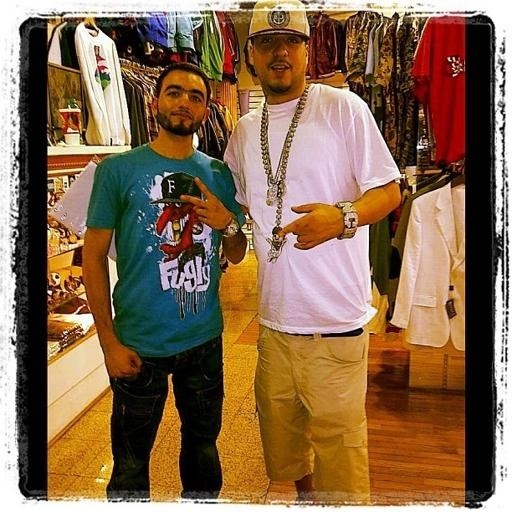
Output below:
[270,325,364,341]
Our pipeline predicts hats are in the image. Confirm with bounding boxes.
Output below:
[247,0,310,41]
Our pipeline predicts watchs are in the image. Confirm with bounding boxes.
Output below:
[218,219,242,237]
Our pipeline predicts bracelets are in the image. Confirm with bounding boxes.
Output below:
[336,202,358,241]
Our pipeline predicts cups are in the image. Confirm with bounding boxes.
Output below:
[64,132,81,146]
[59,109,84,141]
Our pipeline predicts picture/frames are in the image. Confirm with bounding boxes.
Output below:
[47,61,89,147]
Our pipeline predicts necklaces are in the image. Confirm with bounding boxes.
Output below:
[259,83,312,265]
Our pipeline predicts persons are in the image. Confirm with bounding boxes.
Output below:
[219,1,405,503]
[80,62,248,503]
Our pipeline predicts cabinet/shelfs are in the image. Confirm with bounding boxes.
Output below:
[46,146,132,448]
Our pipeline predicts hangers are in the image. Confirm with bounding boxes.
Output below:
[440,159,464,190]
[54,15,99,37]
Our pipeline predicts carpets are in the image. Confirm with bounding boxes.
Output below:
[46,343,270,500]
